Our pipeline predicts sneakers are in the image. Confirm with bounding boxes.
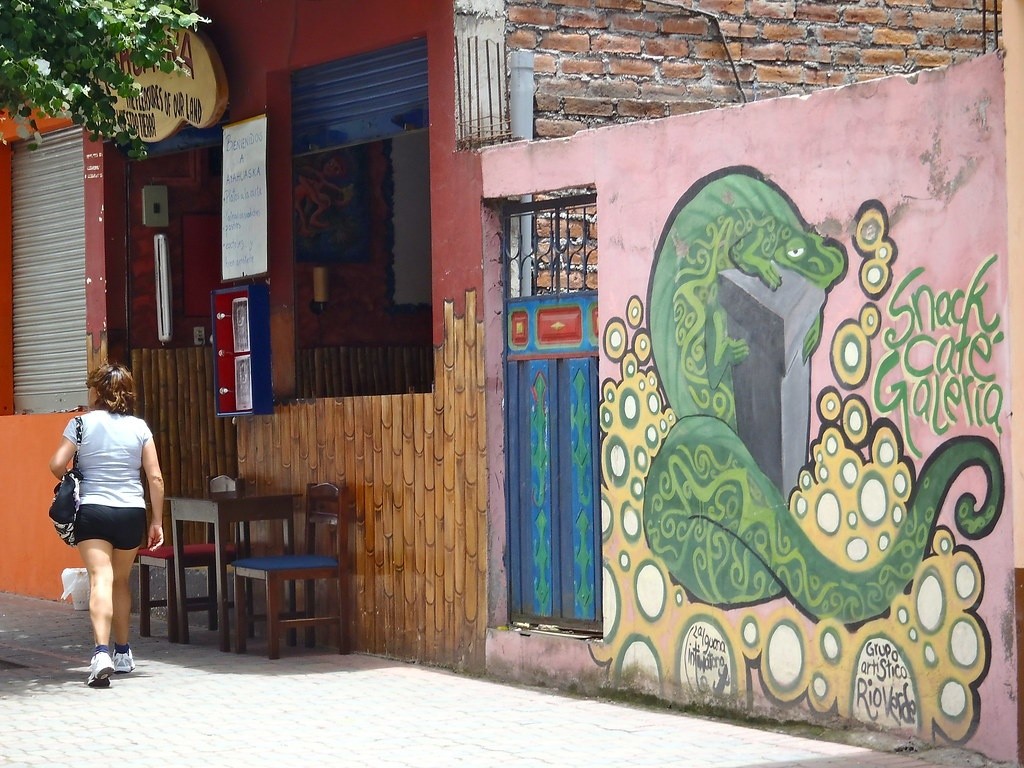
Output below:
[87,649,114,687]
[113,647,135,673]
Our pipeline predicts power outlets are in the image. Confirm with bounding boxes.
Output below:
[194,326,205,345]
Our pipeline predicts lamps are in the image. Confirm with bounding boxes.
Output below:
[153,233,174,343]
[310,266,329,316]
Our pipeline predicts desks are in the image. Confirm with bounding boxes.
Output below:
[165,489,305,654]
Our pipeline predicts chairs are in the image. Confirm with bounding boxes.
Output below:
[134,474,245,645]
[231,481,351,660]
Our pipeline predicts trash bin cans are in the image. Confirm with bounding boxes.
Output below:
[67,568,90,610]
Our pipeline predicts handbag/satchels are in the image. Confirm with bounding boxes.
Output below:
[49,415,83,546]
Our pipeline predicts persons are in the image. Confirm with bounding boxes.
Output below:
[49,366,164,685]
